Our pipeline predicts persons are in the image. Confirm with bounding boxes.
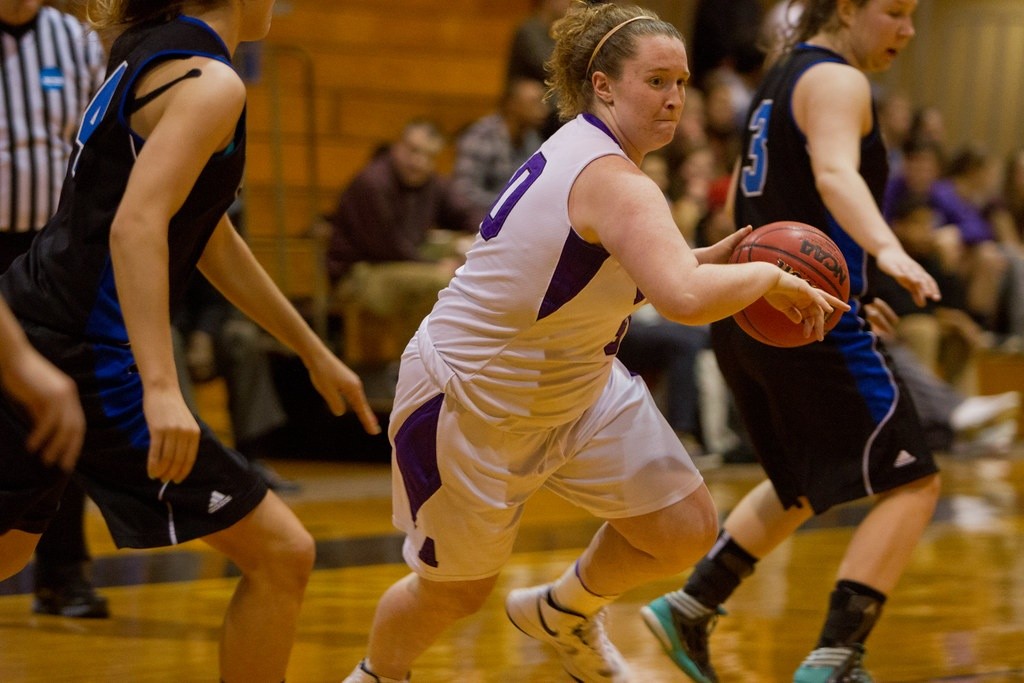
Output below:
[0,0,380,683]
[325,1,569,289]
[638,0,941,683]
[340,0,852,683]
[640,66,1024,462]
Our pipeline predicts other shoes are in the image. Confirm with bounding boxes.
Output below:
[35,585,108,617]
[950,392,1018,455]
[722,446,760,465]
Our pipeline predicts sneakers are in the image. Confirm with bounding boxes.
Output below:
[640,588,728,683]
[341,656,412,683]
[793,643,874,683]
[506,583,633,683]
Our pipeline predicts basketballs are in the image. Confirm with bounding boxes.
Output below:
[726,219,850,349]
